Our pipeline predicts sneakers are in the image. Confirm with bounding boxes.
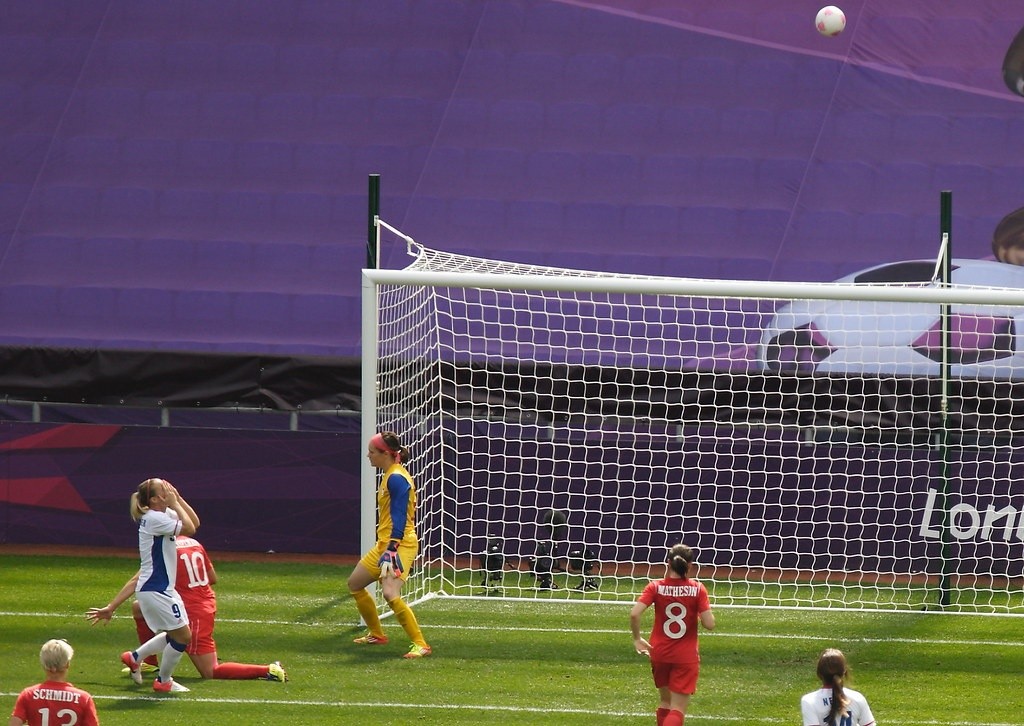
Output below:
[402,640,432,658]
[269,661,287,683]
[353,633,388,644]
[122,650,143,685]
[153,678,190,692]
[123,660,160,672]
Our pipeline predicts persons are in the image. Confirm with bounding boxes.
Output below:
[348,431,432,658]
[800,649,876,726]
[630,544,715,726]
[8,639,100,726]
[85,478,289,692]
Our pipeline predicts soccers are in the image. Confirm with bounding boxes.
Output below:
[756,259,1024,384]
[814,5,846,37]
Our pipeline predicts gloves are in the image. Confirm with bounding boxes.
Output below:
[376,540,404,579]
